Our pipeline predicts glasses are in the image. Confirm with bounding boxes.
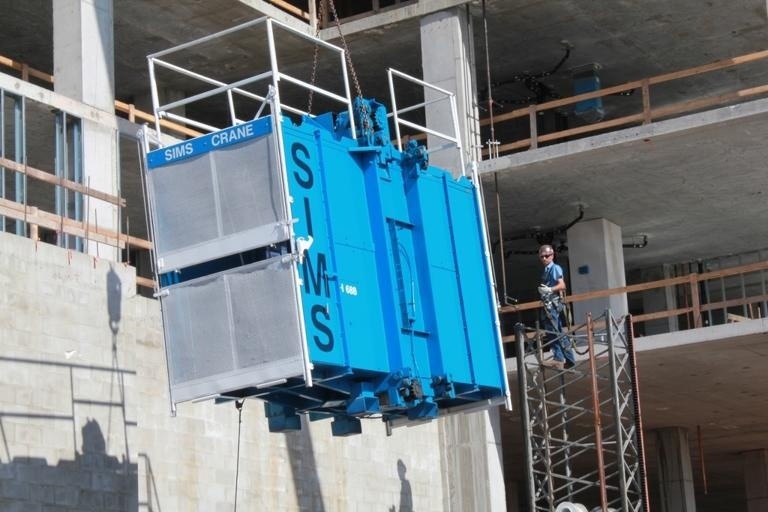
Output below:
[541,253,553,259]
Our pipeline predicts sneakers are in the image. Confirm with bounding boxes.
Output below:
[540,360,564,368]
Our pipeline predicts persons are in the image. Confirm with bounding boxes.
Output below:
[538,245,575,369]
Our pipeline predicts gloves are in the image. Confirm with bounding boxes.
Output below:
[537,284,551,294]
